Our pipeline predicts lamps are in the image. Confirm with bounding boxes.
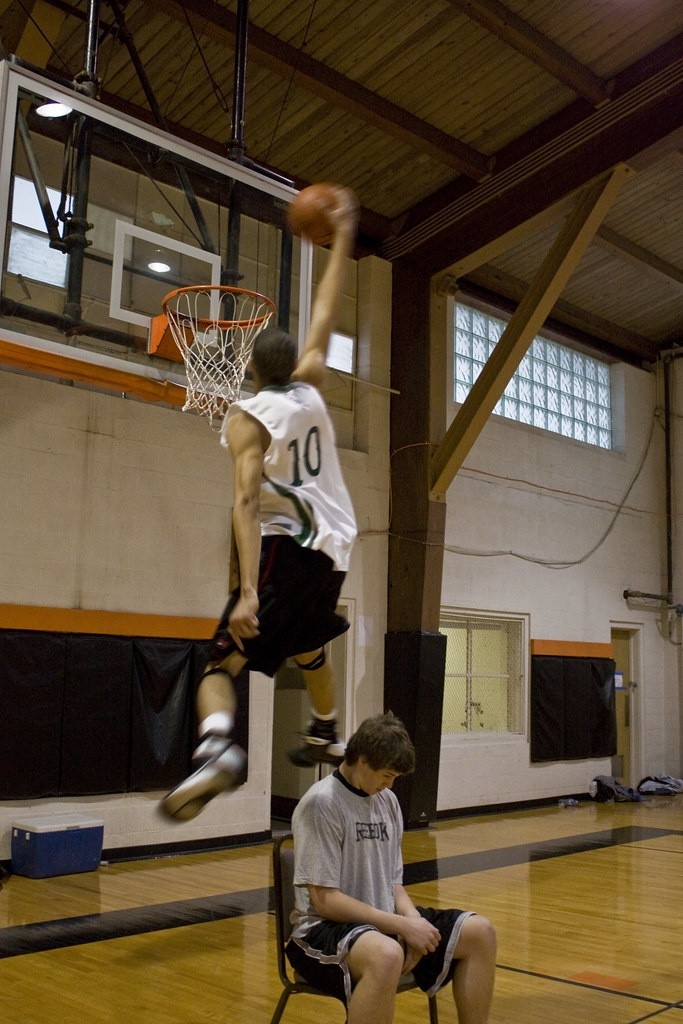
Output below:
[35,98,73,119]
[146,245,171,275]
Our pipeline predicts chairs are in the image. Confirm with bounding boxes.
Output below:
[271,832,439,1024]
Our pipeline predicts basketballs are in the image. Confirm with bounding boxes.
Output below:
[282,186,349,247]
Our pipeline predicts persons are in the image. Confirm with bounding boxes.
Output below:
[161,188,357,822]
[283,710,496,1024]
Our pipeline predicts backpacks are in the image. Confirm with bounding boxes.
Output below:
[592,775,641,804]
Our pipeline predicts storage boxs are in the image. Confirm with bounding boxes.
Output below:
[10,814,105,880]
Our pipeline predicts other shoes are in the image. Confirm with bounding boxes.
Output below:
[284,737,347,768]
[161,739,246,820]
